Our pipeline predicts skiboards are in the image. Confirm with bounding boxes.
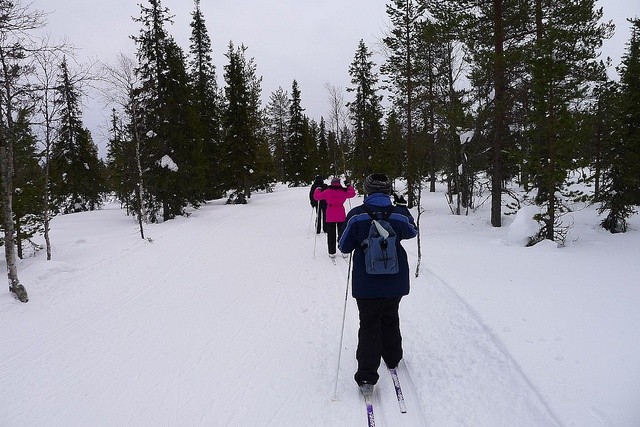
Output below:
[365,368,407,427]
[331,258,349,265]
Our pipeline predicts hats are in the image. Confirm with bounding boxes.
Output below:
[363,172,393,196]
[331,177,341,186]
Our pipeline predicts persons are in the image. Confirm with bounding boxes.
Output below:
[337,172,418,397]
[313,178,356,258]
[309,176,329,234]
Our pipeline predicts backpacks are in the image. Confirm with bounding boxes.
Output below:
[361,204,400,276]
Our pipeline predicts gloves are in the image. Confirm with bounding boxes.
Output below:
[344,180,351,186]
